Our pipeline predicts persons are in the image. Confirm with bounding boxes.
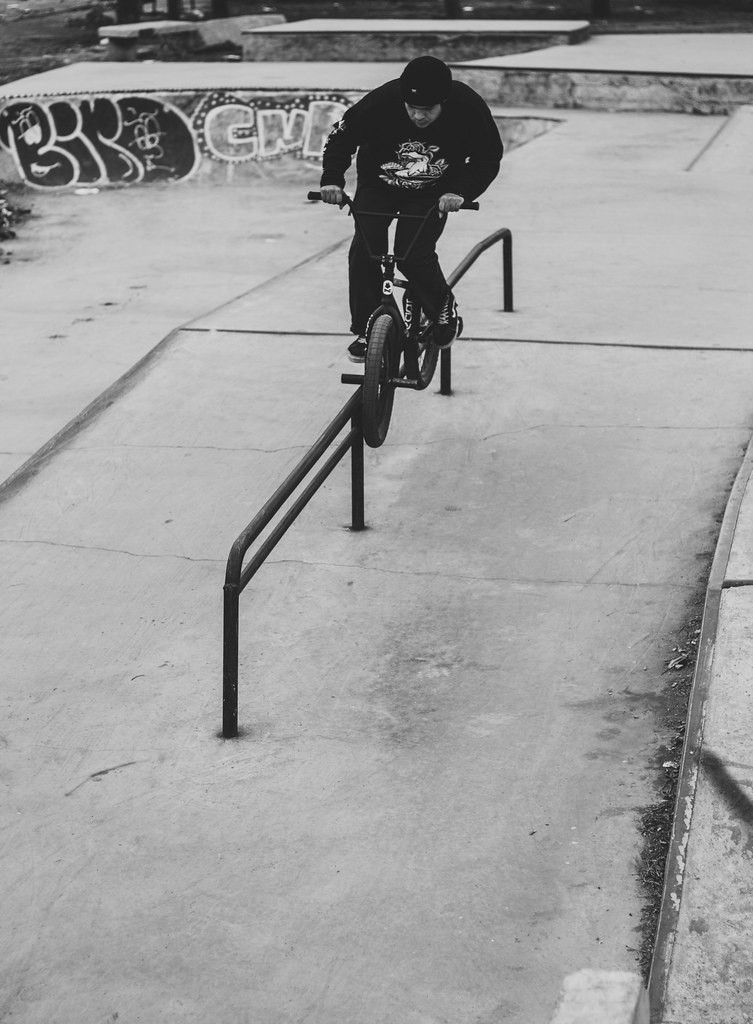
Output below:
[320,55,503,364]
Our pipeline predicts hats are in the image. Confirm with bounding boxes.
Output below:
[400,56,452,105]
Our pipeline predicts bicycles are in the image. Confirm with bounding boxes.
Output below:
[307,190,479,447]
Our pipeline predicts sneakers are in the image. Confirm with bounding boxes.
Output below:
[347,335,368,363]
[433,291,458,348]
